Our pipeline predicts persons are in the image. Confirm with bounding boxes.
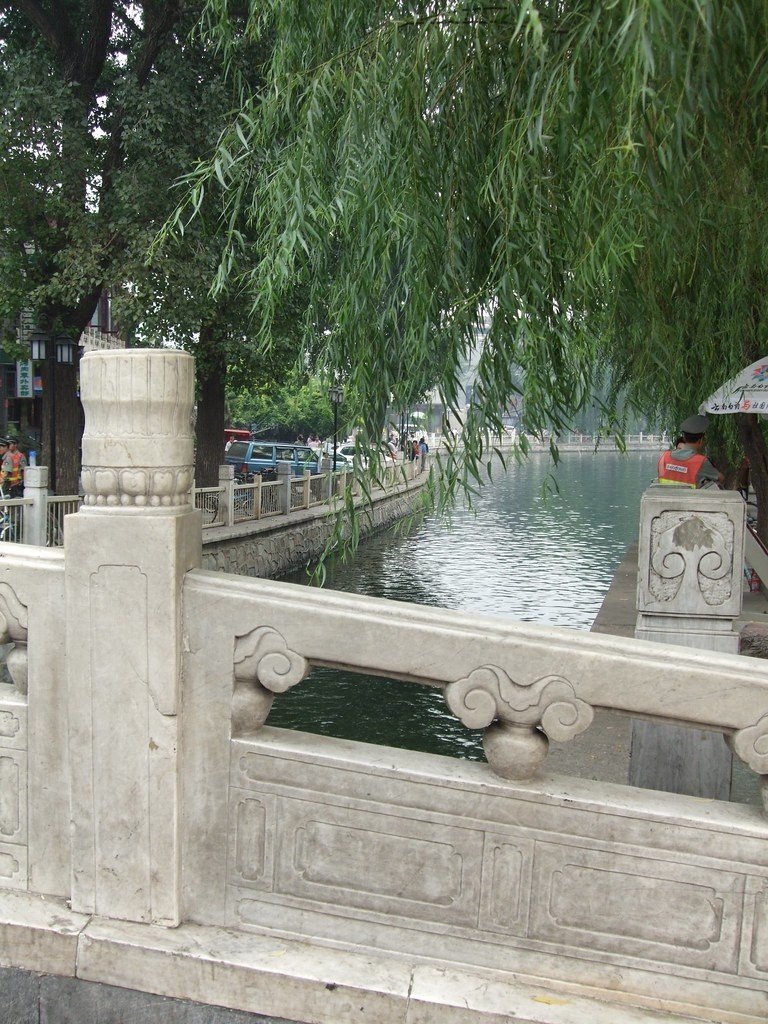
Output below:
[735,453,750,500]
[0,438,19,499]
[675,437,686,450]
[294,433,321,450]
[659,415,725,490]
[5,435,28,497]
[225,434,237,454]
[387,434,428,461]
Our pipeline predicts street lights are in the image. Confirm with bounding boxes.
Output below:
[328,382,344,471]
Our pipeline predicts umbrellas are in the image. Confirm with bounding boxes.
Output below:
[33,376,79,399]
[697,355,767,414]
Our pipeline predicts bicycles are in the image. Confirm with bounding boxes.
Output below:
[196,468,280,524]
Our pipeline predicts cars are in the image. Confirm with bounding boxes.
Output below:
[336,445,396,468]
[312,447,354,471]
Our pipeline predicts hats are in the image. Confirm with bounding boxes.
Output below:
[680,415,710,434]
[6,436,18,443]
[0,437,9,446]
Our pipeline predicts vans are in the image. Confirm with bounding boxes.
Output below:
[397,423,427,438]
[226,440,322,481]
